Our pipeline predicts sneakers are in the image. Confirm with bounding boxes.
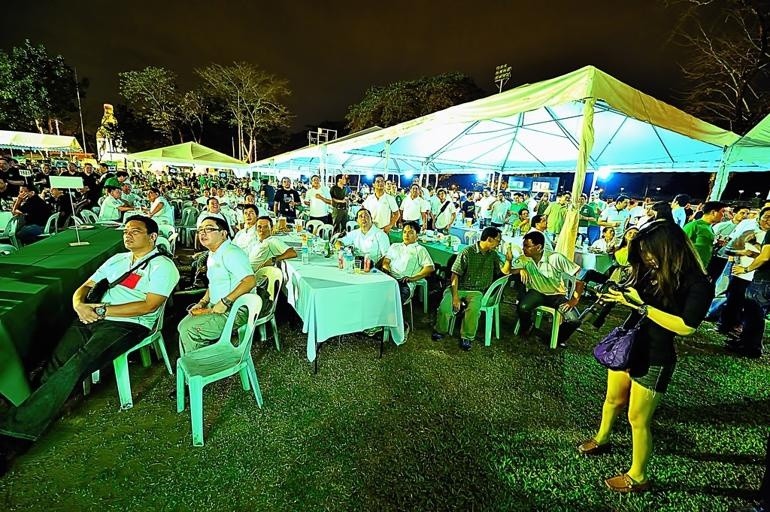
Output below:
[54,392,83,421]
[715,324,720,331]
[725,338,763,357]
[400,321,410,345]
[0,436,15,467]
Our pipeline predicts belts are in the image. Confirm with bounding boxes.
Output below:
[464,286,471,290]
[380,228,385,229]
[355,256,364,259]
[403,220,418,223]
[37,224,41,226]
[337,207,346,210]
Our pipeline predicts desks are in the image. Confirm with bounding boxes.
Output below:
[0,221,129,369]
[388,227,518,265]
[449,224,613,273]
[271,221,404,375]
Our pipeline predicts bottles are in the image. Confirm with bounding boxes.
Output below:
[507,242,512,250]
[346,247,353,271]
[307,235,313,255]
[515,225,520,238]
[452,300,467,315]
[338,247,344,269]
[576,234,582,245]
[433,228,438,239]
[582,237,589,253]
[363,254,370,272]
[301,240,308,265]
[443,236,447,246]
[324,236,330,258]
[479,218,483,229]
[276,218,280,230]
[421,228,427,244]
[446,238,450,249]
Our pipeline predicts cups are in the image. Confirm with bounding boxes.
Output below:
[354,260,361,273]
[279,216,286,228]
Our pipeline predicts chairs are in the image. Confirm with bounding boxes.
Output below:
[176,292,264,447]
[0,210,21,248]
[514,275,576,349]
[80,209,99,224]
[156,237,171,252]
[238,265,284,352]
[71,216,83,225]
[448,273,513,346]
[383,299,413,342]
[37,212,60,236]
[83,299,172,410]
[0,244,17,256]
[158,225,175,257]
[306,220,325,235]
[316,224,334,240]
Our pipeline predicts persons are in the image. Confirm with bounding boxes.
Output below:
[238,178,242,187]
[429,185,443,230]
[598,195,631,239]
[206,175,213,188]
[231,205,259,247]
[259,178,275,209]
[9,159,17,167]
[304,176,333,225]
[80,164,100,192]
[142,189,176,227]
[575,193,597,247]
[242,177,248,188]
[627,199,636,210]
[58,151,68,161]
[274,178,300,222]
[0,179,9,199]
[121,184,142,206]
[94,163,114,194]
[606,197,613,208]
[651,203,675,223]
[97,187,136,221]
[682,201,725,273]
[341,209,390,266]
[579,221,716,493]
[11,184,57,243]
[614,228,640,265]
[362,174,400,235]
[634,217,640,226]
[508,194,529,227]
[491,192,512,227]
[689,203,705,220]
[0,215,181,442]
[329,175,351,233]
[213,175,239,190]
[726,230,770,359]
[452,189,458,205]
[347,184,369,205]
[70,185,96,219]
[390,183,397,196]
[197,197,228,231]
[0,158,25,199]
[195,190,212,206]
[588,192,607,246]
[236,188,244,201]
[293,183,308,194]
[512,209,531,237]
[718,207,770,334]
[528,215,553,251]
[419,189,424,198]
[247,178,251,182]
[705,200,770,322]
[217,187,229,203]
[512,232,585,348]
[199,173,207,187]
[398,184,426,232]
[543,191,571,247]
[101,171,129,196]
[479,186,496,227]
[410,176,431,216]
[177,217,256,355]
[431,227,513,351]
[248,217,298,269]
[242,195,257,207]
[128,170,201,195]
[706,205,751,282]
[201,184,210,195]
[59,163,83,178]
[274,179,280,187]
[33,163,57,185]
[248,177,260,192]
[384,180,392,194]
[209,187,219,199]
[244,194,269,218]
[533,194,551,215]
[364,222,435,346]
[670,194,688,229]
[49,186,77,230]
[522,191,530,204]
[431,190,457,235]
[590,227,618,253]
[685,202,692,225]
[628,201,648,226]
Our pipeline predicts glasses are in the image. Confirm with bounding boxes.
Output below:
[498,195,503,196]
[312,179,319,182]
[146,192,155,194]
[564,195,570,200]
[199,227,219,233]
[514,197,519,199]
[375,180,384,183]
[521,213,528,216]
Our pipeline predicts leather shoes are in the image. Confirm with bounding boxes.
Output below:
[520,321,535,338]
[168,384,189,400]
[432,329,444,341]
[558,341,567,348]
[578,437,612,453]
[461,338,471,350]
[606,473,649,492]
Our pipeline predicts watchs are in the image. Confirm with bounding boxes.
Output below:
[744,266,748,274]
[639,304,649,320]
[220,297,234,307]
[93,305,106,317]
[270,257,277,264]
[504,257,512,264]
[573,291,580,301]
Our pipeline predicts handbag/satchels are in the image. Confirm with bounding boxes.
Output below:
[594,305,647,371]
[434,201,450,229]
[72,253,168,321]
[707,243,722,271]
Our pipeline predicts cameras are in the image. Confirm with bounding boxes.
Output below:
[590,281,623,329]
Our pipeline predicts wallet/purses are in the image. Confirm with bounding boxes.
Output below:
[191,309,213,315]
